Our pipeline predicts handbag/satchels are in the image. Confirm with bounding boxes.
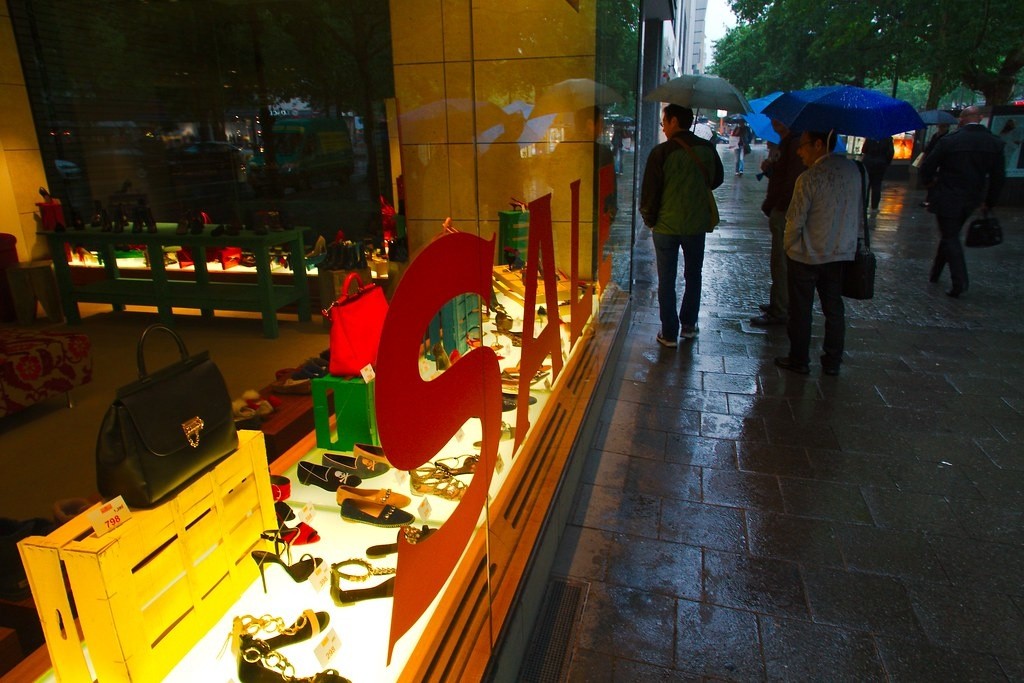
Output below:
[706,186,720,233]
[321,272,390,378]
[744,145,751,154]
[966,209,1003,247]
[96,322,239,510]
[842,252,876,299]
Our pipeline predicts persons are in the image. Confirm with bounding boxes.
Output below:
[637,109,1001,376]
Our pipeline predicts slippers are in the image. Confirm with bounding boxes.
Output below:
[366,525,437,559]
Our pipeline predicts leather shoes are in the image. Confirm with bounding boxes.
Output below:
[775,357,840,375]
[750,304,787,324]
[73,199,157,234]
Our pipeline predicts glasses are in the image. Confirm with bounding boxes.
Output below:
[797,140,811,149]
[659,122,668,127]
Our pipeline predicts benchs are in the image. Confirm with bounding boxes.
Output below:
[0,327,95,418]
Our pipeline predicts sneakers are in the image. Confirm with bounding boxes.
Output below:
[657,327,699,347]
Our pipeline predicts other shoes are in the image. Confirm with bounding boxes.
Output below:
[231,347,330,429]
[176,210,294,236]
[498,358,551,412]
[920,201,931,207]
[735,172,743,176]
[0,496,87,602]
[930,270,960,298]
[297,443,415,528]
[871,208,878,214]
[616,172,623,175]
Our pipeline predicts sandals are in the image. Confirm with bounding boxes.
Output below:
[231,558,396,683]
[270,475,321,545]
[409,421,516,500]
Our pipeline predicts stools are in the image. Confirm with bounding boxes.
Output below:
[0,232,19,322]
[5,259,64,326]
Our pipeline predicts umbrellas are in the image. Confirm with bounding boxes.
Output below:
[643,74,959,161]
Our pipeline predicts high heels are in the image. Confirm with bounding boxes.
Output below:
[251,528,323,593]
[504,246,561,287]
[432,287,548,371]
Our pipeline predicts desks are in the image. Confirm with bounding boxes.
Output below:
[36,220,311,339]
